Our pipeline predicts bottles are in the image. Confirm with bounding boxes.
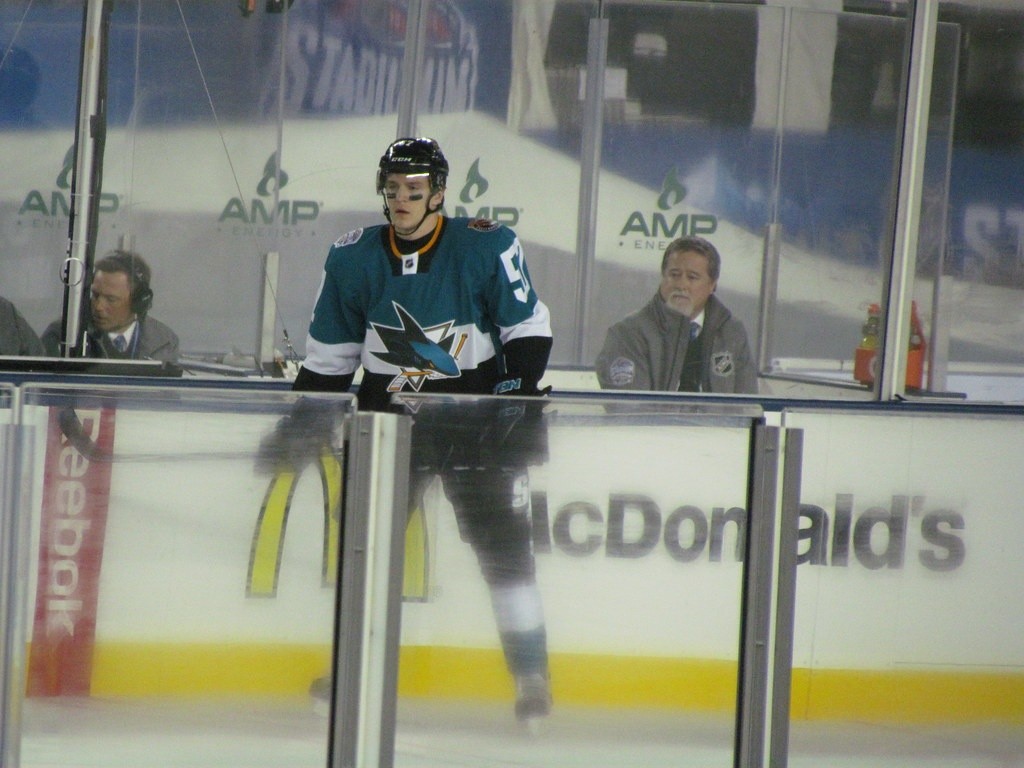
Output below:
[855,302,924,392]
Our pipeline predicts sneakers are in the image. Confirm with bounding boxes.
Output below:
[311,675,334,716]
[515,686,552,740]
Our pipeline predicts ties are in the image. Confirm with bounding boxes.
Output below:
[688,323,699,342]
[114,335,126,352]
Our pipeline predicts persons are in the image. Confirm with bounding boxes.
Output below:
[0,296,45,356]
[40,250,183,377]
[596,236,759,414]
[253,137,553,739]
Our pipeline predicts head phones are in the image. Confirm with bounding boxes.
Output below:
[106,252,153,313]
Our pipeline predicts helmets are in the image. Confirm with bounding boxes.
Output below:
[377,138,449,194]
